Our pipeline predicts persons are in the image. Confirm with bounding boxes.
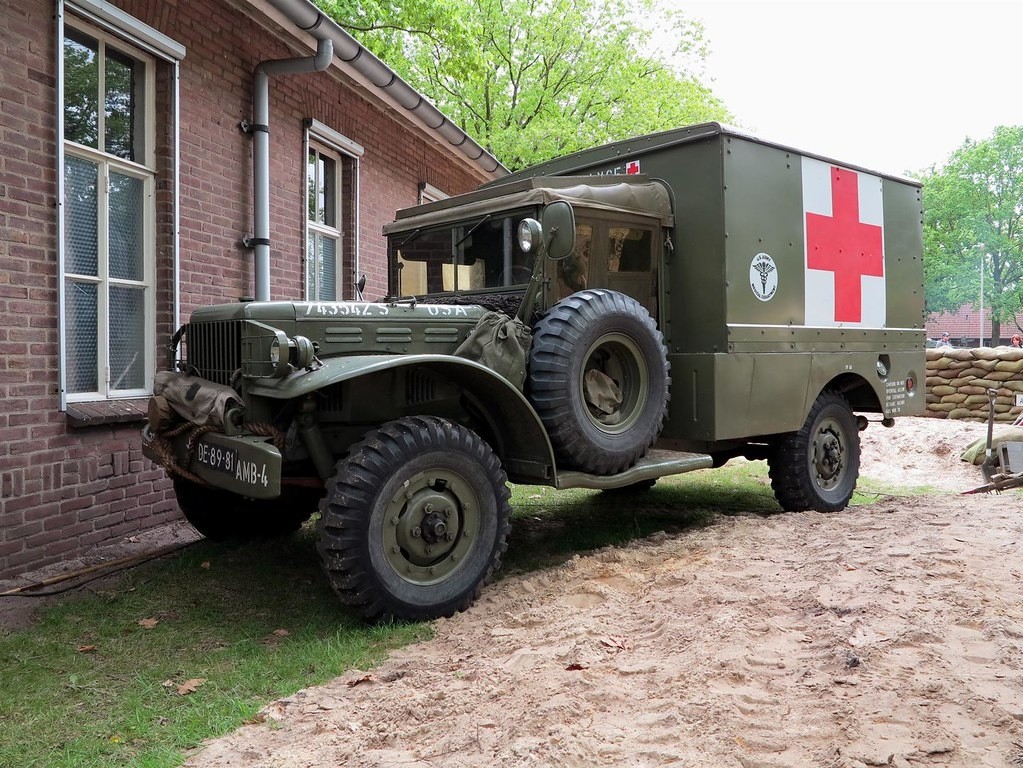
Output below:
[1009,334,1023,348]
[935,332,952,349]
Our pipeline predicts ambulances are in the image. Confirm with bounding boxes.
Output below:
[140,121,926,630]
